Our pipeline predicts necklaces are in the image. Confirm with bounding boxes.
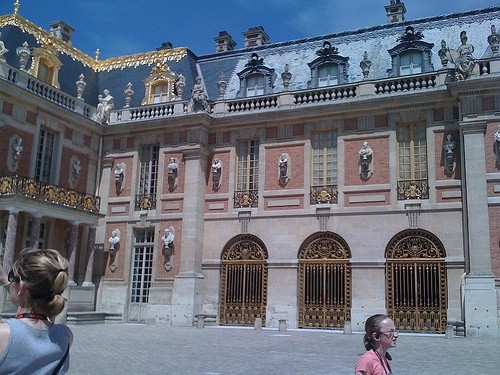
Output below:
[374,350,393,375]
[15,313,47,320]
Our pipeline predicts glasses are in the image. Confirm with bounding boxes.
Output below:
[8,269,21,283]
[379,328,399,334]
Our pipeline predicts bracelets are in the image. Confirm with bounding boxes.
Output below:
[1,281,12,287]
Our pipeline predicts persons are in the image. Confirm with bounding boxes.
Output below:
[0,25,500,249]
[354,314,399,375]
[0,246,74,375]
